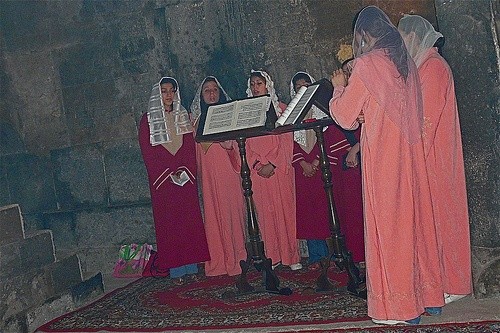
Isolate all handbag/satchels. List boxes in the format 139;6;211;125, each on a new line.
113;244;152;277
142;250;170;277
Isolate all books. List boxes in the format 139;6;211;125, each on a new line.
202;96;271;135
275;84;321;128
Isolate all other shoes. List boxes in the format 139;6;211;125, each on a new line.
172;273;207;285
372;307;441;325
359;261;366;269
308;261;337;269
290;263;302;270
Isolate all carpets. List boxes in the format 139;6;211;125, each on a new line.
266;320;500;333
32;260;433;333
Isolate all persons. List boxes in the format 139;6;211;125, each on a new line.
188;75;250;276
139;77;211;286
244;70;303;271
287;5;474;326
